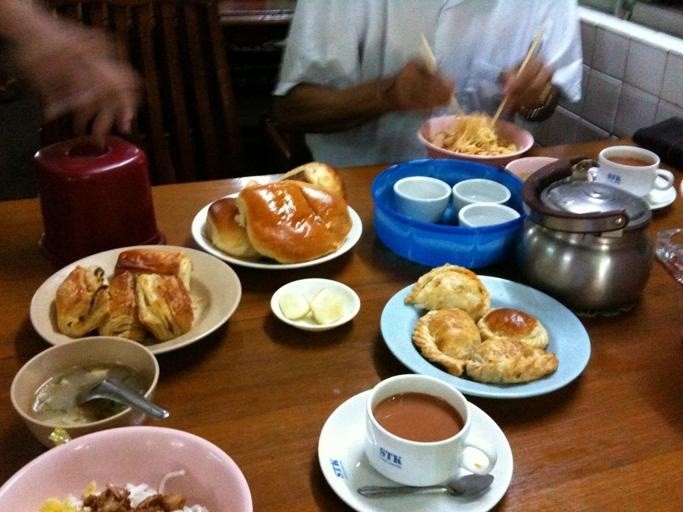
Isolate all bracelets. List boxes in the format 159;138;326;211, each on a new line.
519;86;559;122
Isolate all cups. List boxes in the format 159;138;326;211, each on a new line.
597;144;676;199
364;373;499;489
457;202;520;231
452;177;517;219
391;170;452;224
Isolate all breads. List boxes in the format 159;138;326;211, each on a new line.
57;249;195;345
204;162;353;263
405;263;558;385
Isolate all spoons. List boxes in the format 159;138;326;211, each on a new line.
71;376;170;421
354;474;496;500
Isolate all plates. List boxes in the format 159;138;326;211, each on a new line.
190;191;363;270
269;276;363;333
585;165;678;211
0;425;255;512
379;272;592;401
29;244;242;354
316;388;514;512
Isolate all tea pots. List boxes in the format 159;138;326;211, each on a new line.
514;156;657;321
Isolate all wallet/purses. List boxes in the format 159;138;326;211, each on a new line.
632;116;683;169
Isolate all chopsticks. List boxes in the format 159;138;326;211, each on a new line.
414;35;461;112
488;24;547;131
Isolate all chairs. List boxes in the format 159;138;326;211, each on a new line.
265;41;305;168
45;2;240;183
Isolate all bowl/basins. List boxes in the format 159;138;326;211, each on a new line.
368;158;532;272
7;337;161;449
417;114;537;166
506;157;558;181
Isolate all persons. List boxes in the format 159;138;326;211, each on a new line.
0;0;141;156
270;0;583;169
